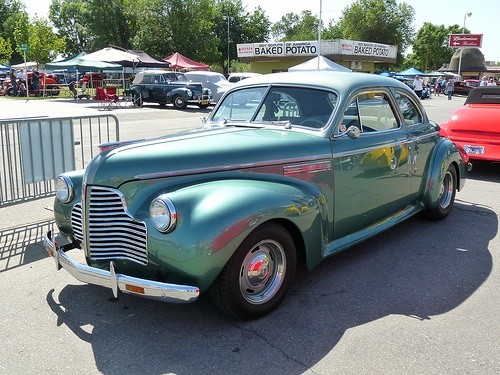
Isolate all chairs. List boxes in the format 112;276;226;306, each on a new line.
278;115;388;134
94;87;122;110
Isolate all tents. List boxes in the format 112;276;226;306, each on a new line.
375;67;461;83
0;45;209;102
287;55;352;73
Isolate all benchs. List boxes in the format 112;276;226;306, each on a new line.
40;88;70;91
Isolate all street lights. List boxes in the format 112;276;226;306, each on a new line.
457;11;473;74
221;15;234;73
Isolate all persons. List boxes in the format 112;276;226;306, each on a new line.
480;76;488;87
425;75;455;101
18;68;26;89
31;67;40;96
10;68;18;96
413;75;424;99
487;79;497;86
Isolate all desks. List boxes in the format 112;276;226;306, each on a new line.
36;84;77;99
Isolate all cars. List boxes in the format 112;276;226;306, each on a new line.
453;77;500;97
208;213;259;257
360;143;410;172
177;71;235;105
42;69;470;321
285;196;328;216
228;73;266;99
439;86;500;165
3;73;61;96
129;70;213;110
